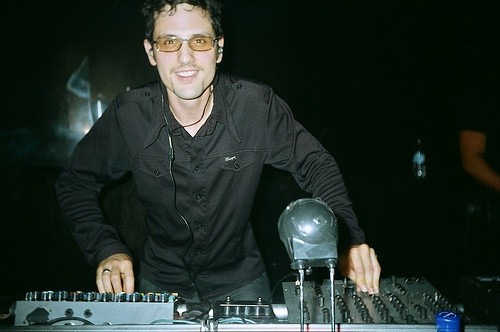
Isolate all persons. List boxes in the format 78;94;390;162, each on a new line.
53;0;381;319
459;71;500;325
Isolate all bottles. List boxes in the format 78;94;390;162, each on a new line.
412;139;426;178
436;312;459;332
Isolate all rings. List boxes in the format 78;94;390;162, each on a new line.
103;269;112;273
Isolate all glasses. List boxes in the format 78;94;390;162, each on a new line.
152;34;220;53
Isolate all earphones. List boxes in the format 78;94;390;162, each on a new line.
149;49;153;56
217;47;223;54
